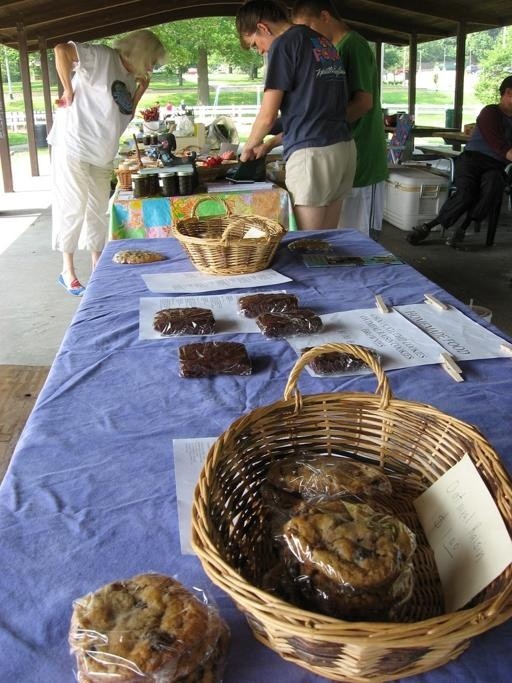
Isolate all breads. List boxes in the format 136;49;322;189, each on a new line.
288;239;329;250
301;345;381;373
262;455;415;621
238;293;298;318
256;308;322;338
154;307;216;335
113;250;166;263
71;574;231;682
178;342;253;377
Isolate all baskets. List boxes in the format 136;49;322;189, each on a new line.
113;158;158;191
171;196;287;276
188;343;511;683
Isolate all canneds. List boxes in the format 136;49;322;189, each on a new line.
143;134;157;145
131;164;193;197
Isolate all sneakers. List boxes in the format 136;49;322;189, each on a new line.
58;272;86;297
404;224;430;245
445;226;465;246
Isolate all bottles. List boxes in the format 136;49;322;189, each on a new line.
143;134;157;145
131;164;194;198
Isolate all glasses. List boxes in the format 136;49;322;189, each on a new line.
250;28;259;51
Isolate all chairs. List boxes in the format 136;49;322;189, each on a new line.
463;122;510;247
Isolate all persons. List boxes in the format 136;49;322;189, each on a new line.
404;76;512;251
291;0;390;239
46;30;166;296
236;0;358;231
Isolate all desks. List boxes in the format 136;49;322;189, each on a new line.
0;177;511;679
384;122;470;151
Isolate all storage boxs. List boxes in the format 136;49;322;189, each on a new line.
383;167;451;232
